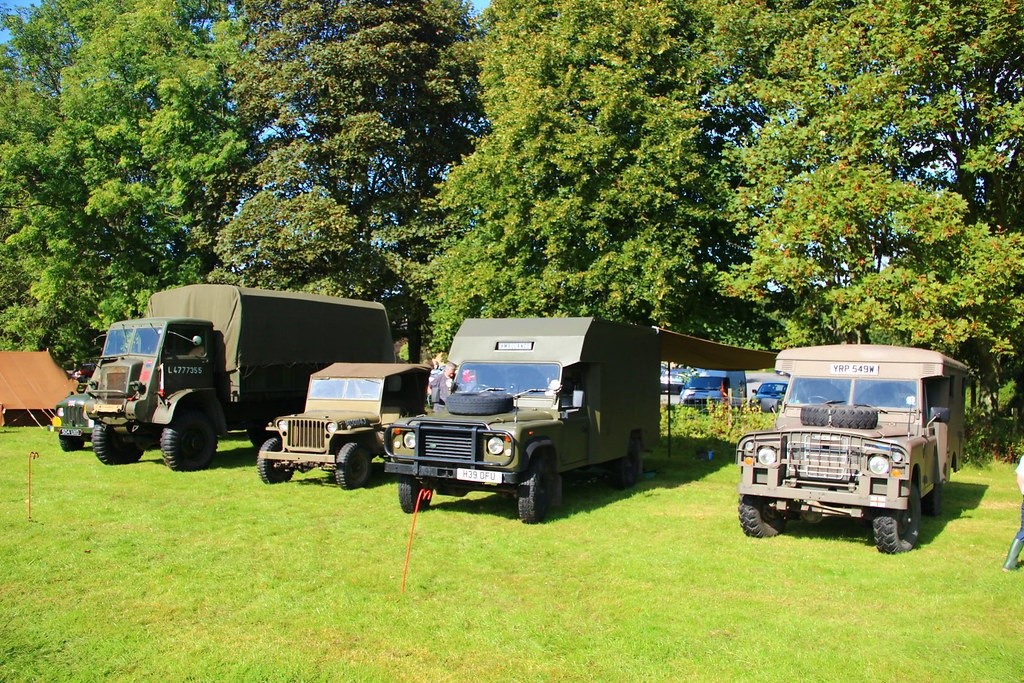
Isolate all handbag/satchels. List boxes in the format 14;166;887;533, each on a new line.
432;381;440;403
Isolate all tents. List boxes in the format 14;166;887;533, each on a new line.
0;348;79;430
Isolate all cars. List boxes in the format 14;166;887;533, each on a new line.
661;363;798;415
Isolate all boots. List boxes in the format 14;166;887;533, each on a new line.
1001;538;1024;573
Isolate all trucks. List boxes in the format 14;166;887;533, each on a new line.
47;282;399;476
51;359;96;451
735;343;972;556
385;314;666;526
258;360;438;491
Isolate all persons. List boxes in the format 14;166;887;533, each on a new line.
427;361;504;411
1001;454;1024;573
